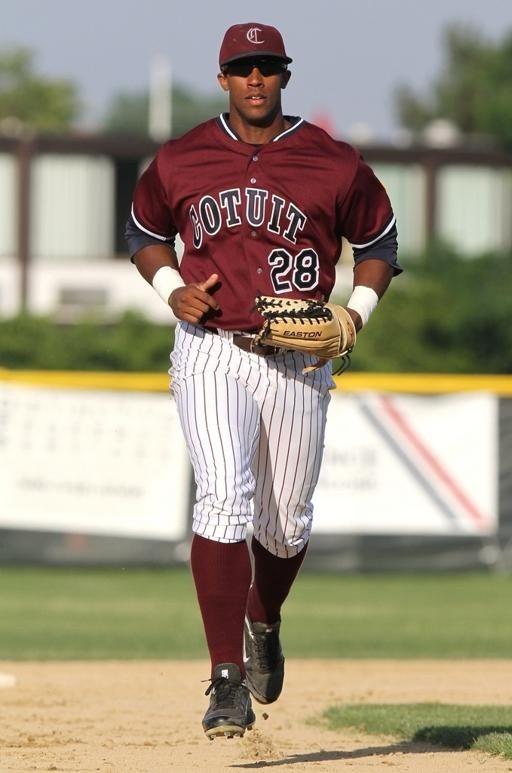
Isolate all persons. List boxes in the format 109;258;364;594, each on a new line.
125;24;404;737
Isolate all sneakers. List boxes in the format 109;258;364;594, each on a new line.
202;663;255;741
244;583;285;704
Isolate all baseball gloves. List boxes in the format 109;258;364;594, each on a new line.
254;295;357;377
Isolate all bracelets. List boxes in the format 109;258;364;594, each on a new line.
348;285;379;327
152;265;186;305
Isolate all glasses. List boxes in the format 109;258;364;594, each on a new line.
223;56;288;77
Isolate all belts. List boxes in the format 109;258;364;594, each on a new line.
199;325;296;357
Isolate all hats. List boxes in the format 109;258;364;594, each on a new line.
219;23;293;67
